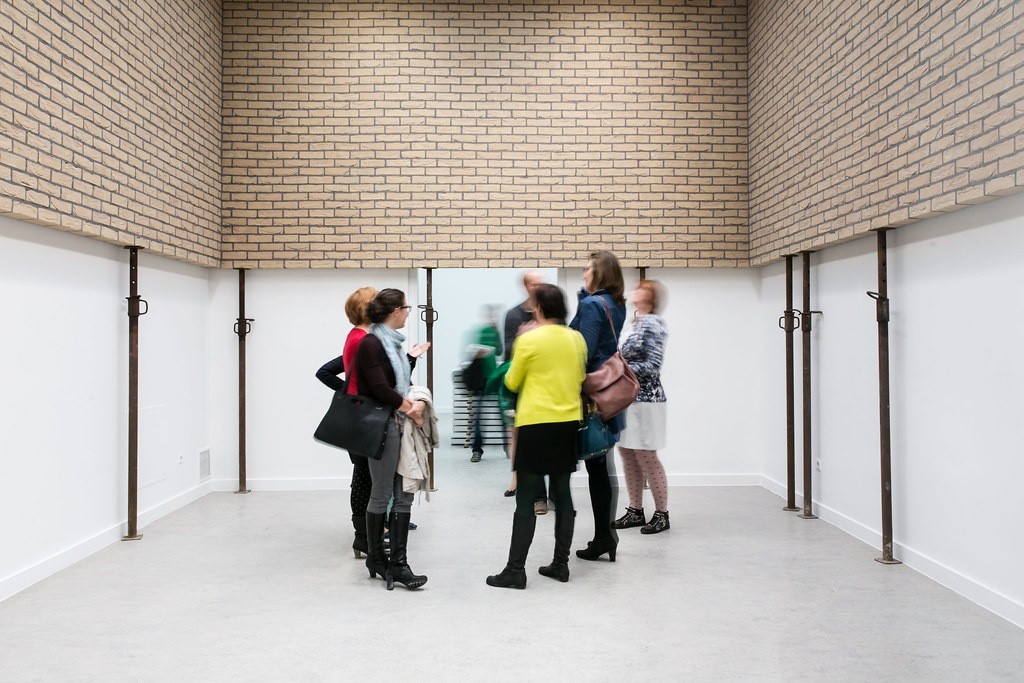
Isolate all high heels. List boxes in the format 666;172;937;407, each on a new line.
575;529;620;563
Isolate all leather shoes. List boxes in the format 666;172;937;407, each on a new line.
505;489;516;496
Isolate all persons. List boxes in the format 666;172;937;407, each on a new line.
486;283;588;588
501;271;555;514
611;279;670;534
356;288;426;590
569;250;617;562
316;287;417;559
469;303;511;463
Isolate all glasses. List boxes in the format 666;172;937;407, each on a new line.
583;266;595;273
389;306;412;314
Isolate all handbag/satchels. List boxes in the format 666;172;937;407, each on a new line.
314;389;394;462
461;357;489;390
583;351;640;422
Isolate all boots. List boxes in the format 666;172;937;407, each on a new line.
386;511;430;590
366;510;390;581
486;513;537;589
353;533;391;559
539;510;576;582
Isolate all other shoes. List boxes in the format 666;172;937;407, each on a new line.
640;510;670;534
534;500;547;514
610;506;646;529
471;452;481;462
